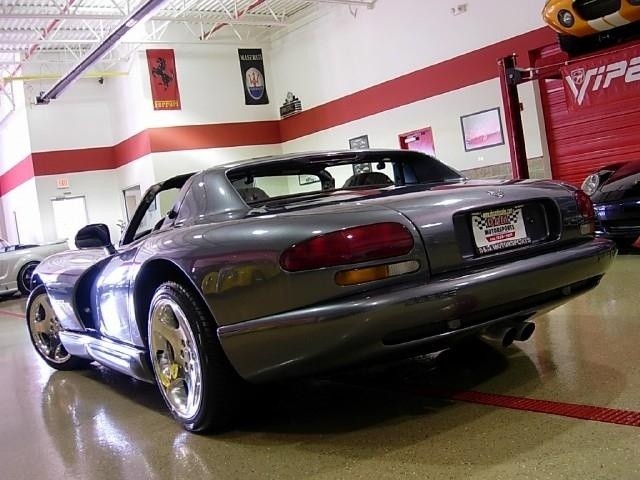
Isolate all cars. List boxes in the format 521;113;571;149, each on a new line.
578;157;638;255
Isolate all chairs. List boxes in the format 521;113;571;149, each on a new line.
235;187;271;206
342;172;396;189
1;245;17;253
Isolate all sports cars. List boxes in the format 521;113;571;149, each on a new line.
0;237;72;300
18;149;606;433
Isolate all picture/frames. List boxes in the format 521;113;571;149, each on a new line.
298;175;320;186
459;106;505;154
348;134;373;176
